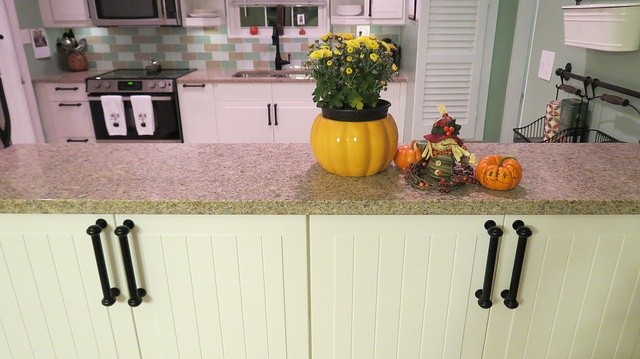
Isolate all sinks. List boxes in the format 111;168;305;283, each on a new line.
233;70;325;80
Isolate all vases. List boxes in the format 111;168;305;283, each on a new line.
312;100;398;176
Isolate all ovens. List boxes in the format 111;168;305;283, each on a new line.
86;93;182;143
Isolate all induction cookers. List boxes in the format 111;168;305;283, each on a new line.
86;68;196;95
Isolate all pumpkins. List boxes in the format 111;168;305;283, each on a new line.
393;143;422;171
475;153;523;191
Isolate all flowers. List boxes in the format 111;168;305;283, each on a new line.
304;32;398;110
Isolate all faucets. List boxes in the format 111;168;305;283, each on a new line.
270;24;291;71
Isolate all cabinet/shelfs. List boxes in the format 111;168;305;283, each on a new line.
180;0;225;27
330;0;405;26
0;214;309;358
39;0;91;28
216;80;321;143
177;83;217;145
309;215;637;358
380;83;406;146
32;82;97;143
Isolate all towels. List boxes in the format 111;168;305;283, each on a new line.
100;95;127;135
130;94;156;137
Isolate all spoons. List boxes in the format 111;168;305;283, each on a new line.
62;36;87;54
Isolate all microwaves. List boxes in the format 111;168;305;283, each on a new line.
88;0;181;27
